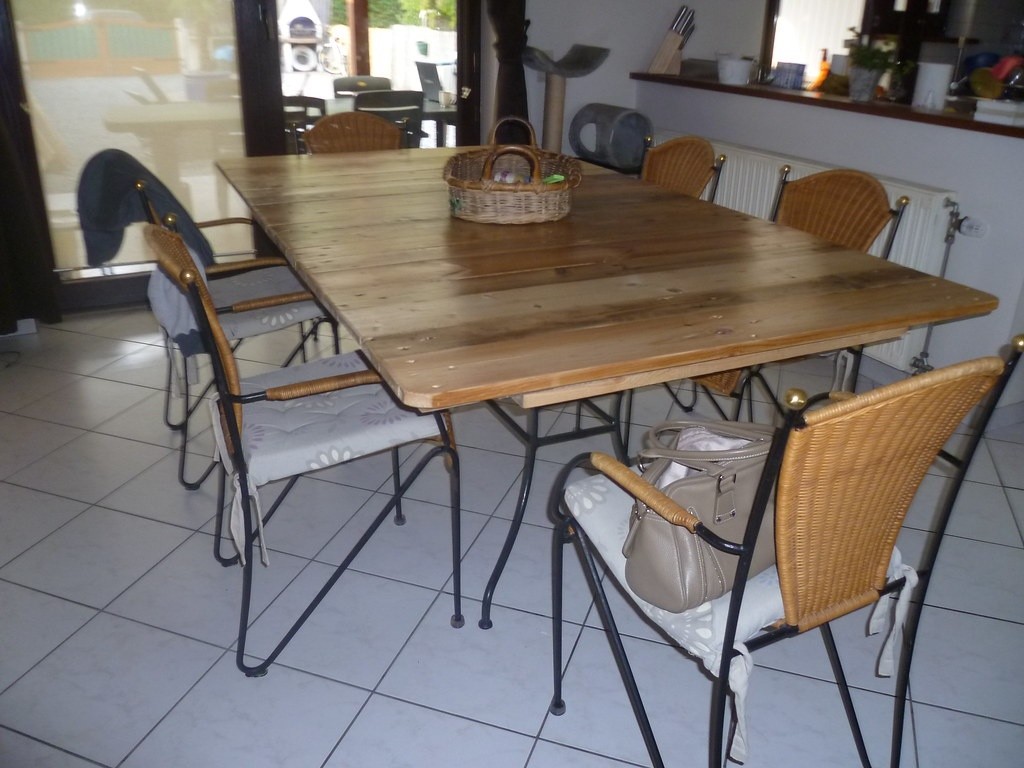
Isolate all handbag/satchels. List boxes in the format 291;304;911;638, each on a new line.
621;420;776;614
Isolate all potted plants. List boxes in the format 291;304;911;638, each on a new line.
849;42;897;103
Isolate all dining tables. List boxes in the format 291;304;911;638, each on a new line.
642;137;910;423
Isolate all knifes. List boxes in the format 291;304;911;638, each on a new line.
669;5;695;51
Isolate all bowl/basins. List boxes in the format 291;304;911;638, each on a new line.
965;51;1024;99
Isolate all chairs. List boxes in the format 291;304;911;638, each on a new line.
75;148;464;679
545;332;1024;768
301;110;403;344
283;76;428;155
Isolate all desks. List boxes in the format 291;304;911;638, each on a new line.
211;144;999;631
284;96;458;148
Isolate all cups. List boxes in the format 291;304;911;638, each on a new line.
438;91;453;108
912;62;954;112
719;57;752;85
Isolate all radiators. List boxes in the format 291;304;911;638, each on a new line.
653;126;987;376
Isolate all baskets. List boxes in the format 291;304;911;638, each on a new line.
444;115;581;225
